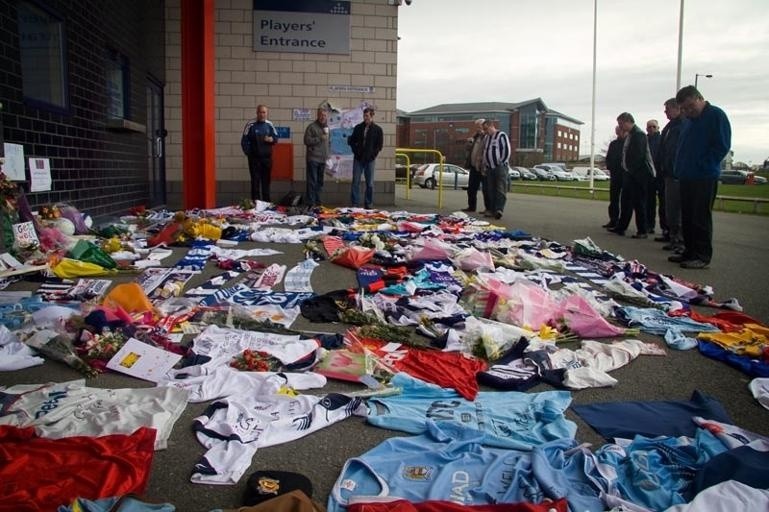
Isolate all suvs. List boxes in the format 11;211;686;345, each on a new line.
533;165;574;181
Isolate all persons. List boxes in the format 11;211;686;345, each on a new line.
241;105;279;202
602;84;731;268
350;108;383;209
460;119;511;219
303;110;330;206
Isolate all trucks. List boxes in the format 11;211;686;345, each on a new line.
573;167;608;181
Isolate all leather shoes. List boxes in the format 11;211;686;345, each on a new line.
654;228;710;269
601;221;654;239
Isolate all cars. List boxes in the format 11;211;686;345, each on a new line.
602;169;611;177
529;168;554;181
409;164;422;179
564;168;590;182
513;167;537;181
694;74;712;90
413;163;470;191
507;166;520;180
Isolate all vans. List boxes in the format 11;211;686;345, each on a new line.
718;170;767;185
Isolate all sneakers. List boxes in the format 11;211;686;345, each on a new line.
462;206;503;219
